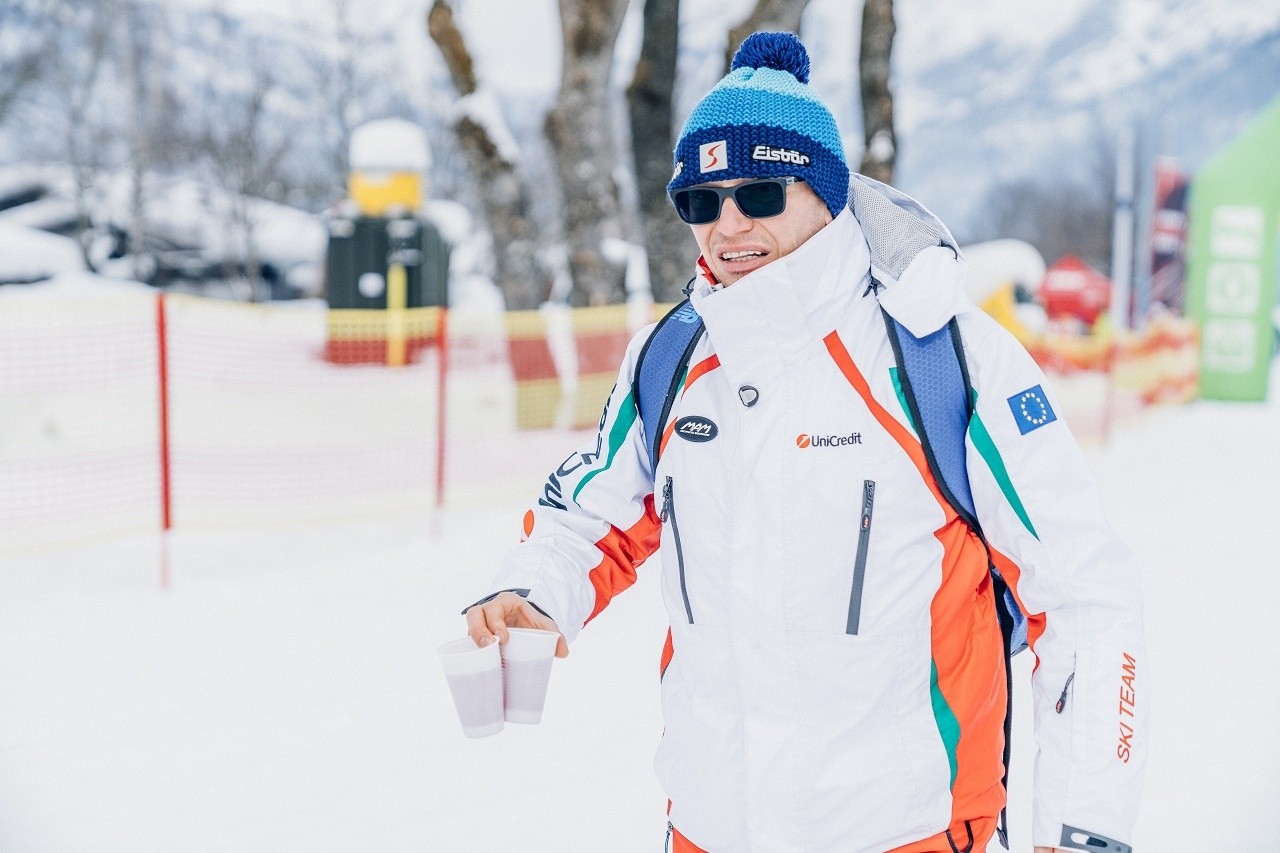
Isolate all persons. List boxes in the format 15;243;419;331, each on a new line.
460;28;1149;852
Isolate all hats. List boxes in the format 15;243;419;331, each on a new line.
666;32;850;219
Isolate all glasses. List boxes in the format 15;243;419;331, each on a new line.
669;173;805;225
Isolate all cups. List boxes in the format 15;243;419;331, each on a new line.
500;625;559;723
436;634;504;737
